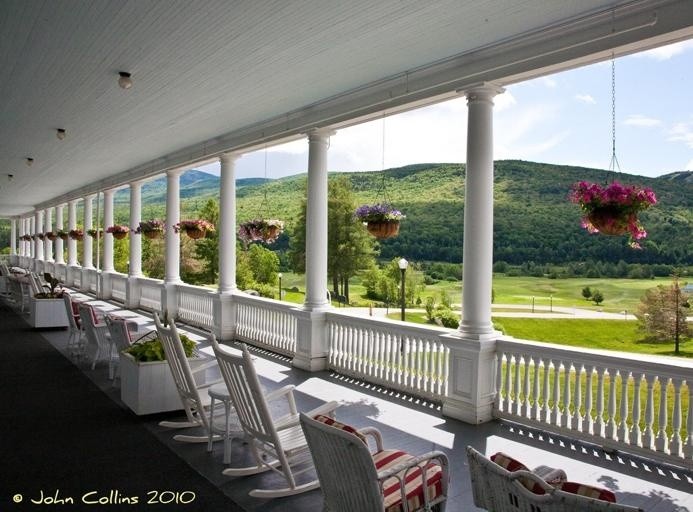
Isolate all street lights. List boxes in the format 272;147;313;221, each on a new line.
279;272;283;300
126;260;130;273
550;294;553;312
532;296;535;313
673;283;681;352
398;258;409;355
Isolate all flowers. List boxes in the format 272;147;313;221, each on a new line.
17;224;129;240
568;175;658;250
238;219;284;245
132;216;165;237
356;203;405;222
173;218;215;232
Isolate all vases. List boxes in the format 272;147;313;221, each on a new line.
143;230;158;238
186;230;205;239
586;216;626;236
367;221;399;238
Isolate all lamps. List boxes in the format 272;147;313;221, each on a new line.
56;128;66;138
25;157;33;167
117;70;131;89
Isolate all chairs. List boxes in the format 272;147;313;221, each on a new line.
0;259;637;512
